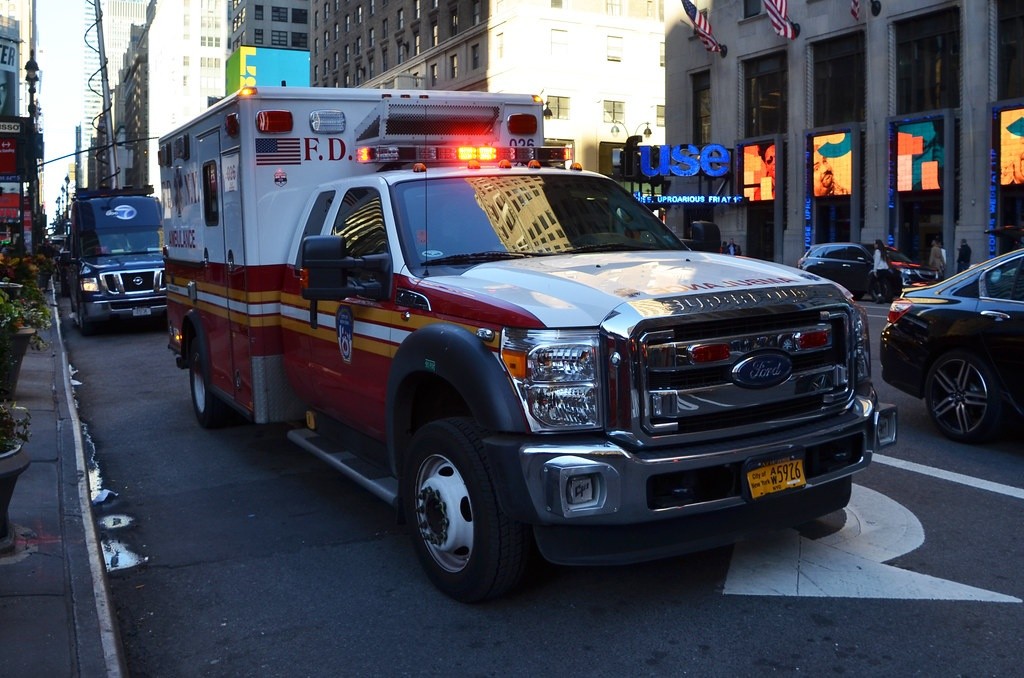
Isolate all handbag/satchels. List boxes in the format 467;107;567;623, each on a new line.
876;287;890;304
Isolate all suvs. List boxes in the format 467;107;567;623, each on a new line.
796;242;944;305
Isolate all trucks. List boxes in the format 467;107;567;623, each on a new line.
70;187;167;336
157;84;900;607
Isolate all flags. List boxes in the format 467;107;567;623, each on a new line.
681;0;725;56
851;0;862;20
765;0;797;41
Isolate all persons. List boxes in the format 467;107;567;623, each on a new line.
957;239;971;274
754;143;777;200
936;240;946;263
929;239;945;273
720;238;742;256
814;145;850;196
873;238;890;304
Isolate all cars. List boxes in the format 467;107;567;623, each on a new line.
880;248;1024;447
